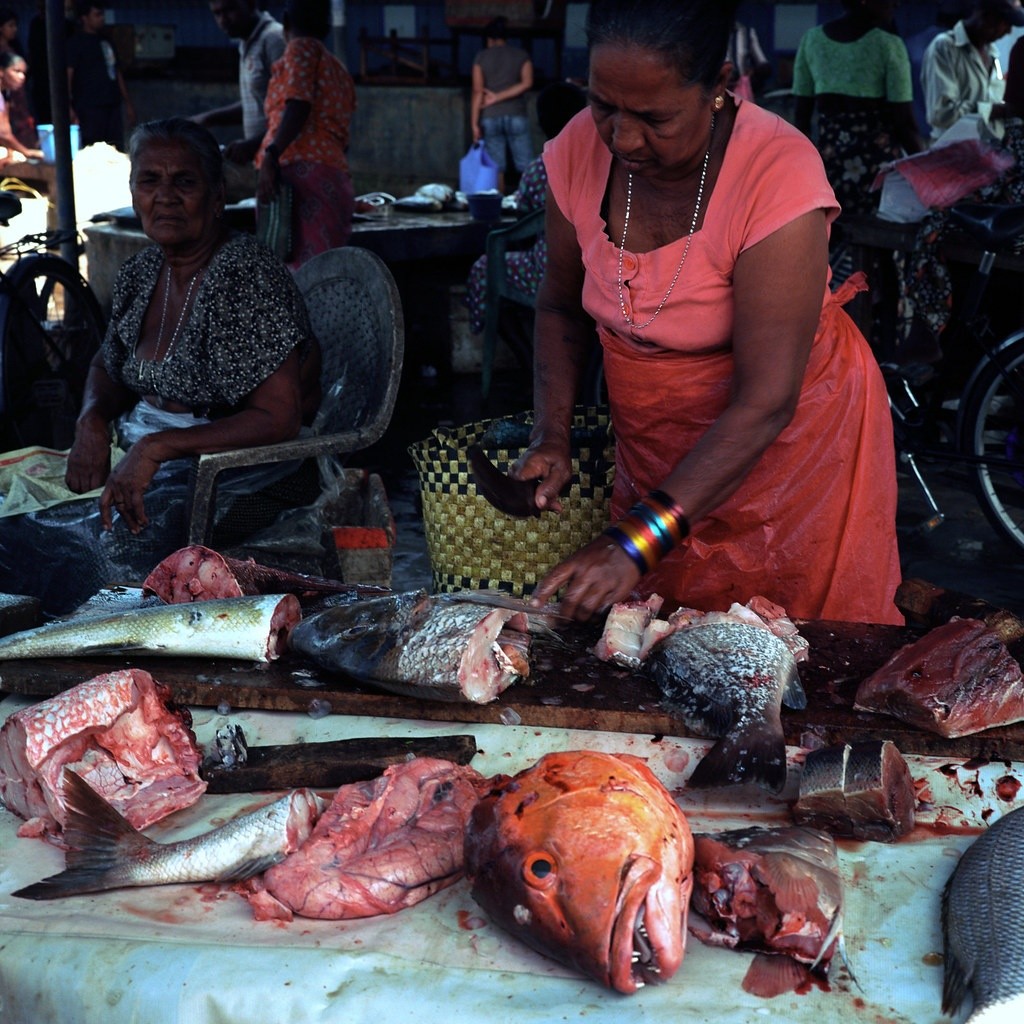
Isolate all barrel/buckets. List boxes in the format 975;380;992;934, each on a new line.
36;123;80;164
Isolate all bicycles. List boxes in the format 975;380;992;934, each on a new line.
937;193;1024;562
0;191;108;453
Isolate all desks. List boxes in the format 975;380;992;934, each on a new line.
840;222;1024;334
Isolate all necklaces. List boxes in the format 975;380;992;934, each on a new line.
618;113;715;329
151;267;202;409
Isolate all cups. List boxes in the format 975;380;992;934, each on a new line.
466;193;503;221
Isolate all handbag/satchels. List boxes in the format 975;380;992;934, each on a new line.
255;180;297;258
459;139;501;195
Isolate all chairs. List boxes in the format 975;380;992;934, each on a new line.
480;205;547;400
187;244;406;550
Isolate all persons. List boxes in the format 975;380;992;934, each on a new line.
466;82;587;336
471;16;534;196
66;0;136;152
184;0;288;163
0;52;45;170
0;117;328;620
790;0;953;365
919;0;1024;145
0;10;34;134
254;0;358;275
506;0;906;630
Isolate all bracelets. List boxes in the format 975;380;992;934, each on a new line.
601;489;690;576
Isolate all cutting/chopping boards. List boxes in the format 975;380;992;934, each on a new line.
0;586;1023;760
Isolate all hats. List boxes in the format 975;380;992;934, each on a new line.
975;0;1024;27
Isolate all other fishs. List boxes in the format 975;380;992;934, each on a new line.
0;542;1024;1024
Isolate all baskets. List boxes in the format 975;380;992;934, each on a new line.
410;405;617;605
